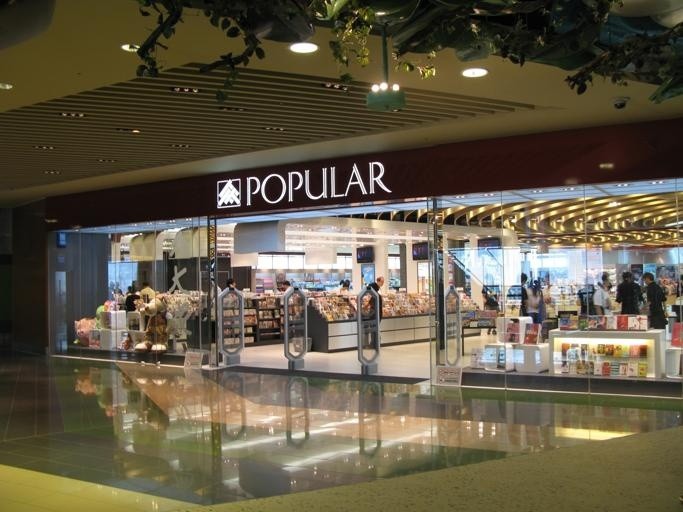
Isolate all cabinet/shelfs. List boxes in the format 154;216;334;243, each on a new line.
309;295;481;349
547;327;668;381
214;298;304;349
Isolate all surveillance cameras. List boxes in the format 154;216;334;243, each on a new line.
611;96;631;109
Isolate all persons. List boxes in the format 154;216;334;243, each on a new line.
107;279;136;302
365;281;382;350
515;271;682;337
124;287;138;311
335;279;352;294
139;280;155;328
225;278;236;287
372;275;385;292
282;281;294;296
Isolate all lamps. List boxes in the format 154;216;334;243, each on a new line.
363;25;405;114
306;246;337;265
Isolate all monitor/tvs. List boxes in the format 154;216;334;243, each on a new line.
477;238;500;257
412;243;428;260
356;247;372;263
